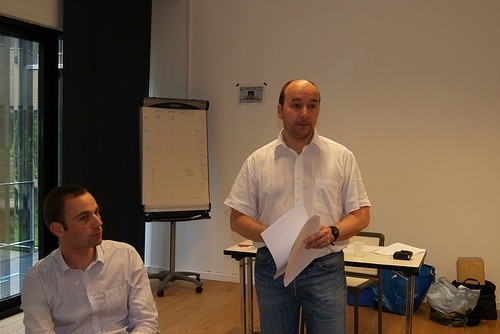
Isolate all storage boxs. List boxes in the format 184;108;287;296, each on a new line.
456;256;486;284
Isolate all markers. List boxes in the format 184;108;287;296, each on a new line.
191;215;201;219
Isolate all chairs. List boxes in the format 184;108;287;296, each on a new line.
301;231;385;334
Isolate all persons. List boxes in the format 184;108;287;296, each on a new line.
20;185;160;334
224;79;370;334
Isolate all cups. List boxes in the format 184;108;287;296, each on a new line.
353;242;364;257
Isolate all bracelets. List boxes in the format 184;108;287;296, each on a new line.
329;225;340;245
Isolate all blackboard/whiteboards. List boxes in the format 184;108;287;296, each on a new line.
136;97;212;217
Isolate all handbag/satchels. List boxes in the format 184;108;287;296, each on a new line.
451;278;498;320
427;275;481;327
371;262;436;316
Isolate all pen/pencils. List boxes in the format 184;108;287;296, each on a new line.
237;244;253;247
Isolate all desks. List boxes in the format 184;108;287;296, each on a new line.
224;239;427;334
